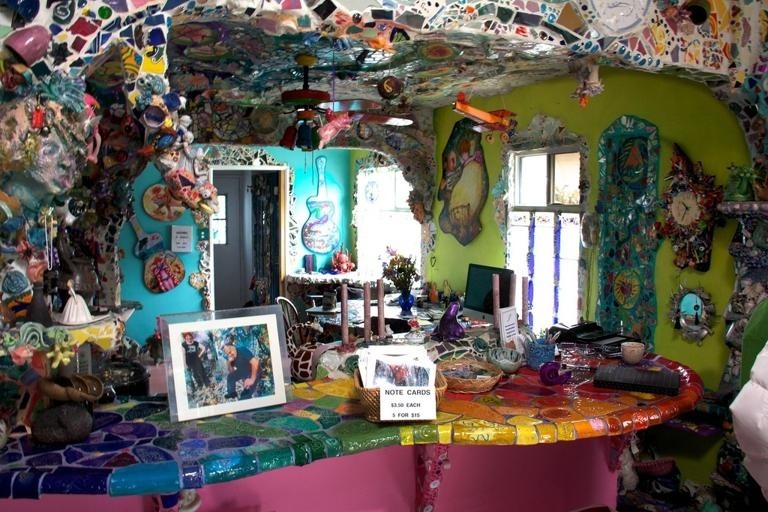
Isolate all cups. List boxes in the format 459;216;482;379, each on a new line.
527;340;555;371
305;254;313;273
620;341;650;365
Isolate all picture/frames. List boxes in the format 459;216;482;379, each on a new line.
157;305;293;423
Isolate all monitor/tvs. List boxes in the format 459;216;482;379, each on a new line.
462;263;514;337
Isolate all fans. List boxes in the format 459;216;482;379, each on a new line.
227;57;413;127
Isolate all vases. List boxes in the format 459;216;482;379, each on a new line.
399;290;414;315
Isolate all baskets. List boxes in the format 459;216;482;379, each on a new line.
353;367;447;424
439;359;503;394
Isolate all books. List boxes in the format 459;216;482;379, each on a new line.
589;363;682;398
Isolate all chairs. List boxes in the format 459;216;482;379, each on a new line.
305;286;365;306
275;296;303;346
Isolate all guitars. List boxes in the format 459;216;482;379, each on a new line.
301;156;341;254
127;203;185;294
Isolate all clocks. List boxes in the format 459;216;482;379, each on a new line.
659;144;721;271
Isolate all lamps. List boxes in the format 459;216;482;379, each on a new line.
281;107;325;151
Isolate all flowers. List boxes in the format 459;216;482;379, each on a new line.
382;244;420;304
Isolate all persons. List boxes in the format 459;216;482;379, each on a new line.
181;332;210;389
223;342;262;399
